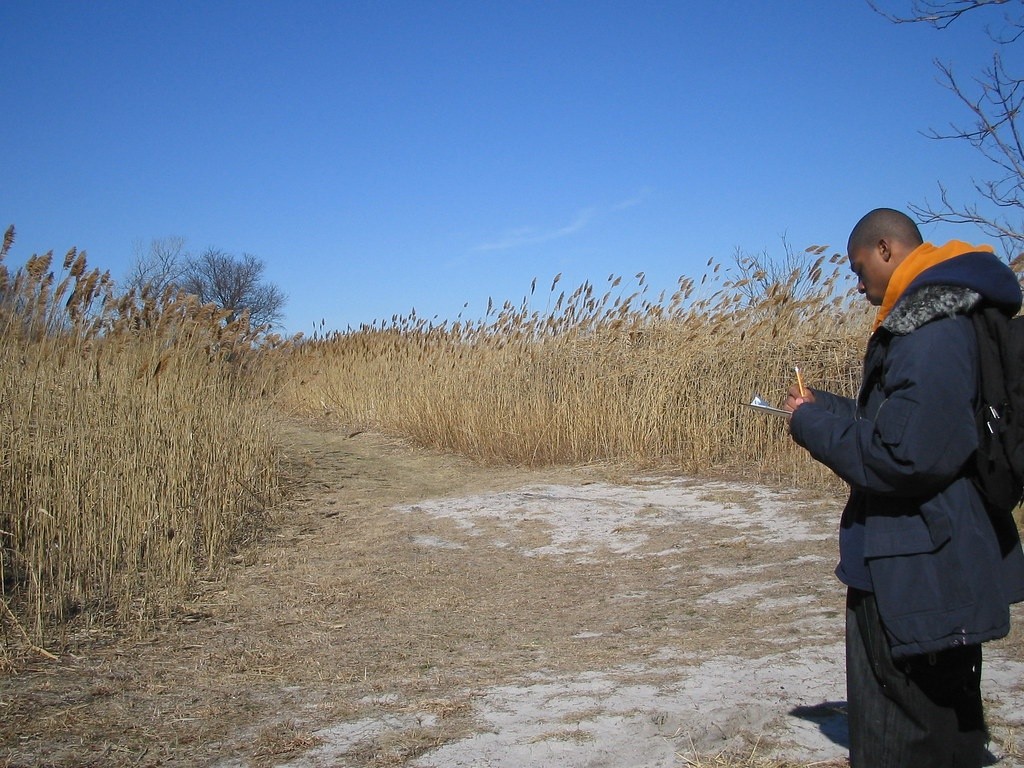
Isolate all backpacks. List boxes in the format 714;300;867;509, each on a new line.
962;299;1024;519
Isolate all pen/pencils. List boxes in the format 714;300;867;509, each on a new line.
794;366;804;397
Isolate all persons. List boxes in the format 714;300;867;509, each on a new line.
781;208;1024;768
